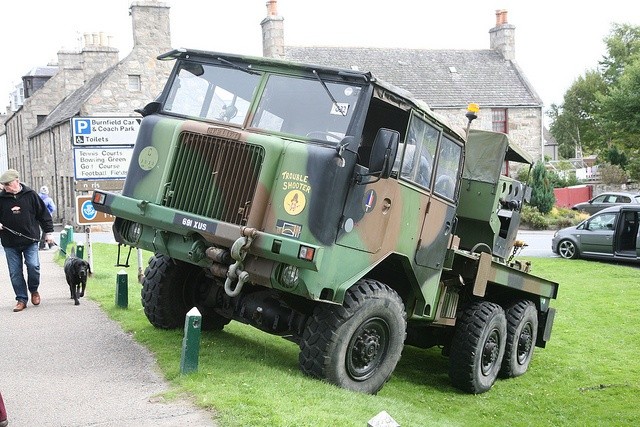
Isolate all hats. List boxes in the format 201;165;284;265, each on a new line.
39;185;50;195
1;169;19;182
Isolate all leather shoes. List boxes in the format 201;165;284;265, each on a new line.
31;290;40;305
12;300;27;312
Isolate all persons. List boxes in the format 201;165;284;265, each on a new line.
34;185;57;251
0;168;57;313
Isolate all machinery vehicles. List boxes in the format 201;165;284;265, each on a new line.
91;48;559;395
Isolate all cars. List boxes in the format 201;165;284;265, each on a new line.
552;205;640;266
571;192;640;215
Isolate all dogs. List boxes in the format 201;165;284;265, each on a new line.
64;254;92;304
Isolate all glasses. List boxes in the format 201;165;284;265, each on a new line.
1;182;11;187
